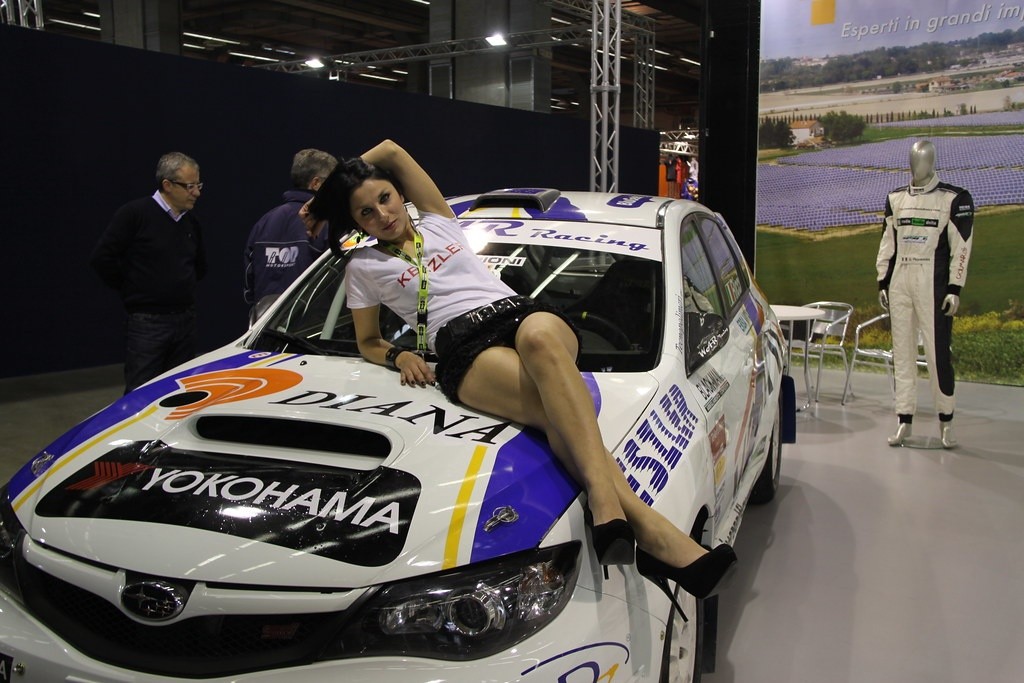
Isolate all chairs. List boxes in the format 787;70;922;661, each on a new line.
841;311;927;405
778;301;857;402
591;258;663;357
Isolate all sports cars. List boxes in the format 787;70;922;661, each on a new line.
0;184;798;683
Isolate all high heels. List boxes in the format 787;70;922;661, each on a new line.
582;499;635;581
634;543;739;622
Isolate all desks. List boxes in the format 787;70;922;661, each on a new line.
769;304;826;411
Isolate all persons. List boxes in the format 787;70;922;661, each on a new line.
875;141;974;447
85;153;207;397
244;150;338;321
299;140;737;599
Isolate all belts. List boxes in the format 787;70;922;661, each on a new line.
446;293;534;342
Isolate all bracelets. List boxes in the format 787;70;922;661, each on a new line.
386;347;412;370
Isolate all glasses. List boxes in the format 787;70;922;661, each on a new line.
160;178;204;191
